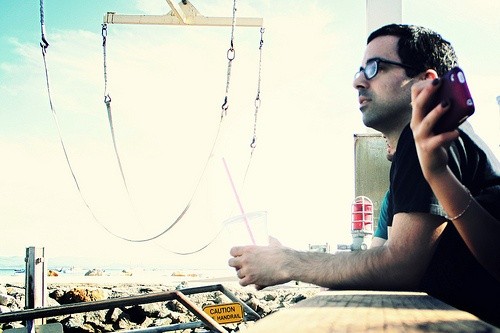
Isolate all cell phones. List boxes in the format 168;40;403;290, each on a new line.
422;66;475;136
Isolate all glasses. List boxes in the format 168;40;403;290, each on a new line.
354;58;406;80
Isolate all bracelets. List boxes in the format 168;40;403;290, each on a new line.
445;192;472;220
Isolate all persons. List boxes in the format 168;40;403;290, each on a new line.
409;78;500;279
228;22;500;328
